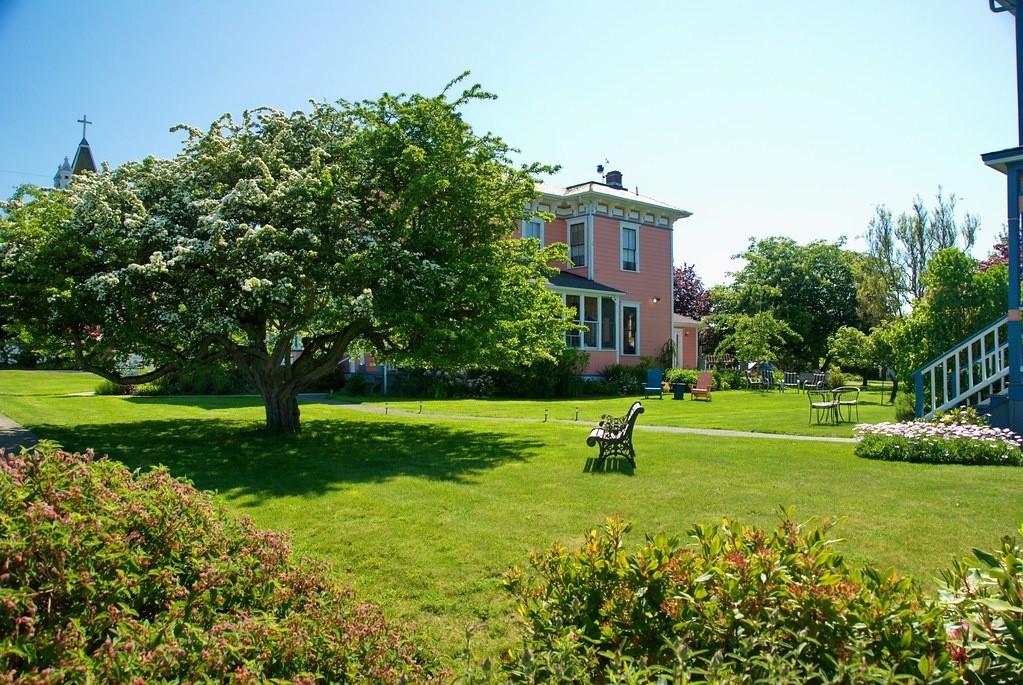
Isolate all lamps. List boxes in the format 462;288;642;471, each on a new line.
653;298;660;303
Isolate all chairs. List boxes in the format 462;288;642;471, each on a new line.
803;373;826;394
689;372;713;401
807;386;860;424
641;368;665;399
761;370;781;393
781;372;800;394
746;370;762;390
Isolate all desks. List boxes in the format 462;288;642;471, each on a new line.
672;383;686;399
811;390;851;426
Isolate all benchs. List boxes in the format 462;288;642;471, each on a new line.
586;402;645;470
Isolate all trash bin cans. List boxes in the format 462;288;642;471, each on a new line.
671;383;686;399
